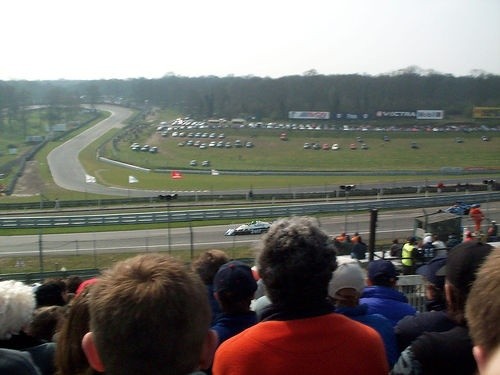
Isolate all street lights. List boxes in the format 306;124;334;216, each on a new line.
483;179;496;234
156;193;178;255
339;184;357;234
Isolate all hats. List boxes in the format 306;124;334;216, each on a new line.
327;261;364;300
464;231;471;235
366;260;400;284
435;240;496;288
406;236;417;241
415;255;450;284
423;236;432;243
214;261;258;304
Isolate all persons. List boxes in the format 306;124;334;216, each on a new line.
326;256;417;369
192;249;227;283
488;221;497;242
391;231;473;292
210;261;258;342
466;246;500;375
394;252;450;352
212;218;390;375
333;232;368;260
0;277;101;375
82;253;218;375
470;204;484;231
391;241;496;375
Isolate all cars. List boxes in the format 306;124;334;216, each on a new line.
224;220;272;236
128;114;500;167
444;201;481;216
